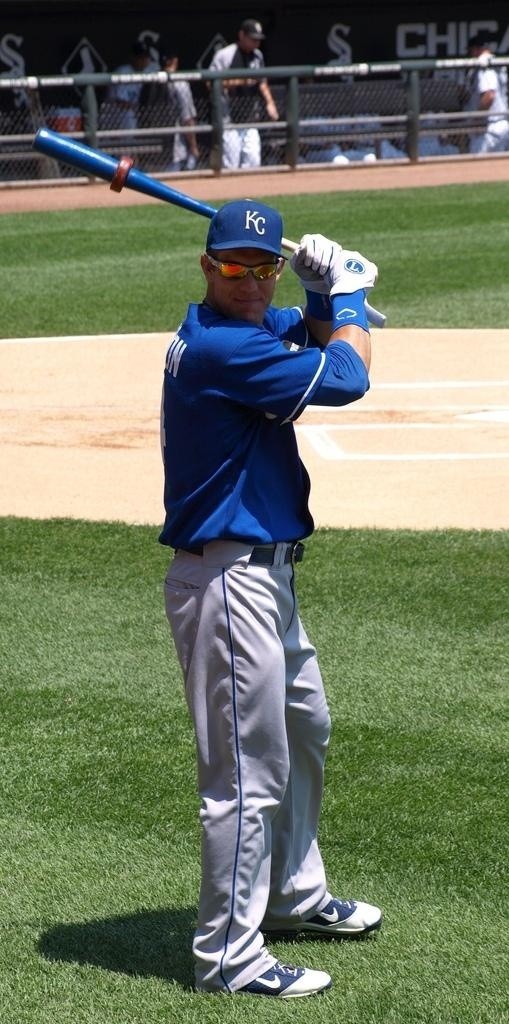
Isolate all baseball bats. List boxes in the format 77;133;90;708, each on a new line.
33;125;298;254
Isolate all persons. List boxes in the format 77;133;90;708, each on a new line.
458;33;508;153
105;42;200;173
206;20;279;169
158;198;384;998
260;107;459;166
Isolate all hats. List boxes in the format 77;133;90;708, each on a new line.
206;200;288;259
242;20;264;39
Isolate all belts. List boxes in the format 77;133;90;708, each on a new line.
182;540;304;564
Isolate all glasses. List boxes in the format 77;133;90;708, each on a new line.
204;253;281;280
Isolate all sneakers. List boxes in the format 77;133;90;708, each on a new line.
236;962;330;998
260;898;381;936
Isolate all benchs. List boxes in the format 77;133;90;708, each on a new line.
100;80;486;165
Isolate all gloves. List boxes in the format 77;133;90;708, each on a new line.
290;234;377;300
